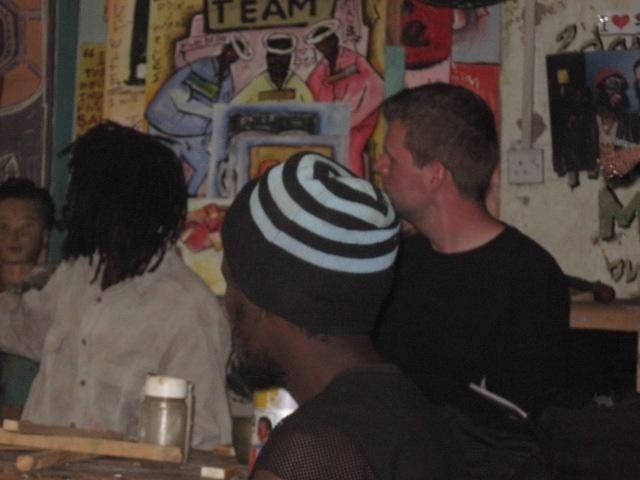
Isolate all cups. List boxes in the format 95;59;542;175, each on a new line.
136;374;188;469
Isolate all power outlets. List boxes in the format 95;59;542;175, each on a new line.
505;148;545;184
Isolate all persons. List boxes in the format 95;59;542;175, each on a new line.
140;32;253;201
303;20;389;183
226;29;318;105
374;78;574;438
1;120;237;457
564;70;638;174
220;148;476;480
1;174;56;414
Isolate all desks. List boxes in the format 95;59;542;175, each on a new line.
566;298;639;393
0;418;251;480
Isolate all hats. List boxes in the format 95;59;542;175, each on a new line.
594;67;628;92
220;151;401;326
